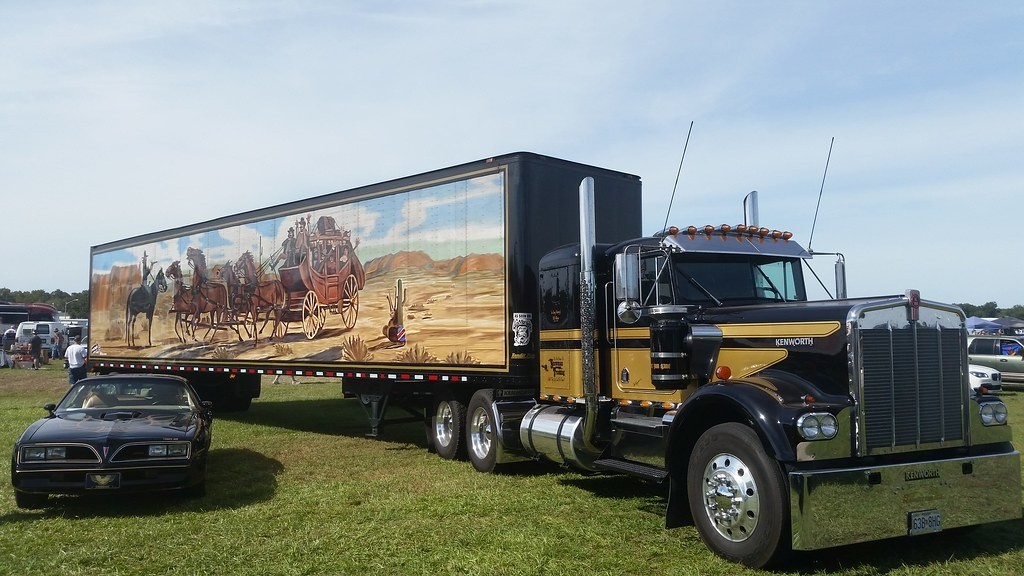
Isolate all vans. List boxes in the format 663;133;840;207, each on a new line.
967;335;1024;384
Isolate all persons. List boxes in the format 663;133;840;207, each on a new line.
28;330;42;370
967;328;1024;337
272;374;301;385
51;328;63;361
63;334;88;385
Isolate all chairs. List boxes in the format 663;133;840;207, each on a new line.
81;393;122;409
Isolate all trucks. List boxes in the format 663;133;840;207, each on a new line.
0;302;59;324
14;319;88;358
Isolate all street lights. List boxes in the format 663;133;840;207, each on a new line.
65;299;78;319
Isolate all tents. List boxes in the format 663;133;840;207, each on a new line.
991;316;1023;330
966;316;1002;337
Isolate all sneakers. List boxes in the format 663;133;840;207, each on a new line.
273;381;281;386
292;381;301;385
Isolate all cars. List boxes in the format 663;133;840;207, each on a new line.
968;364;1002;394
11;373;214;509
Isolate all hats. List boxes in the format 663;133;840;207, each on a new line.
32;330;39;334
53;328;59;331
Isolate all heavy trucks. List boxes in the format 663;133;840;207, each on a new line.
87;120;1024;570
